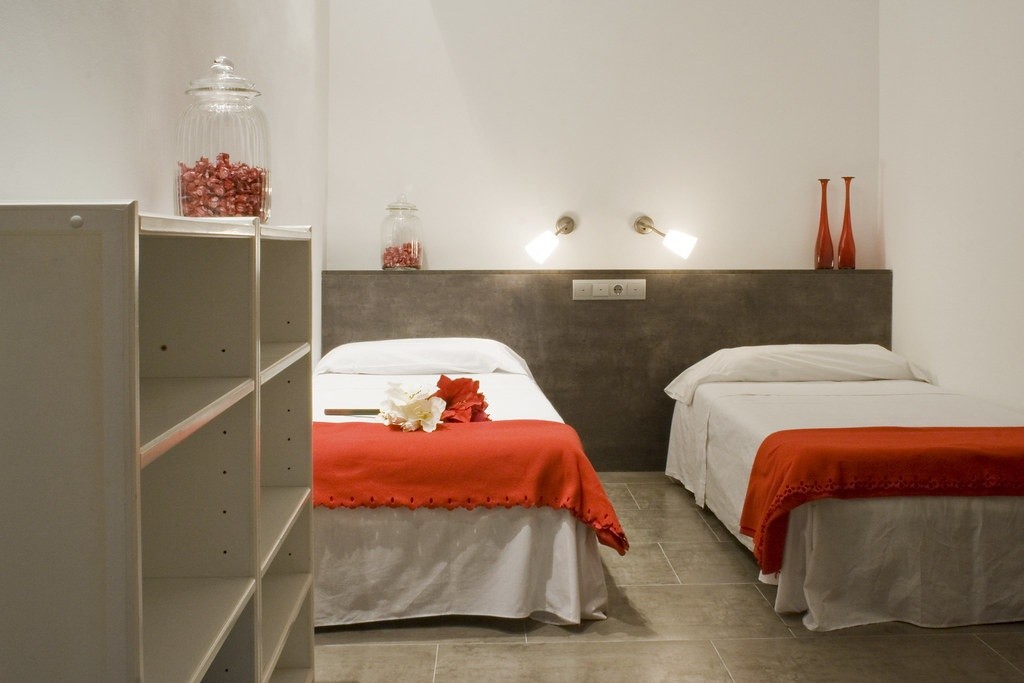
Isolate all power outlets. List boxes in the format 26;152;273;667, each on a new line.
610;279;627;300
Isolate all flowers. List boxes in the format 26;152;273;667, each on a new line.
325;375;492;432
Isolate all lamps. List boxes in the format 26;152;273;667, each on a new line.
634;216;698;260
525;216;574;264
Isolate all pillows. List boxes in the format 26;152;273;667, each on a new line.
313;338;525;377
663;344;932;407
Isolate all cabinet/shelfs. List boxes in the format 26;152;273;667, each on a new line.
0;201;315;683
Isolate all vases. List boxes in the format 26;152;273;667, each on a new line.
814;177;856;269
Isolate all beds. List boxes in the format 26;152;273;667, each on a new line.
313;370;631;626
665;380;1024;633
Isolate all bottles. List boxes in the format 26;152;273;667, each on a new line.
174;56;272;219
378;194;422;270
